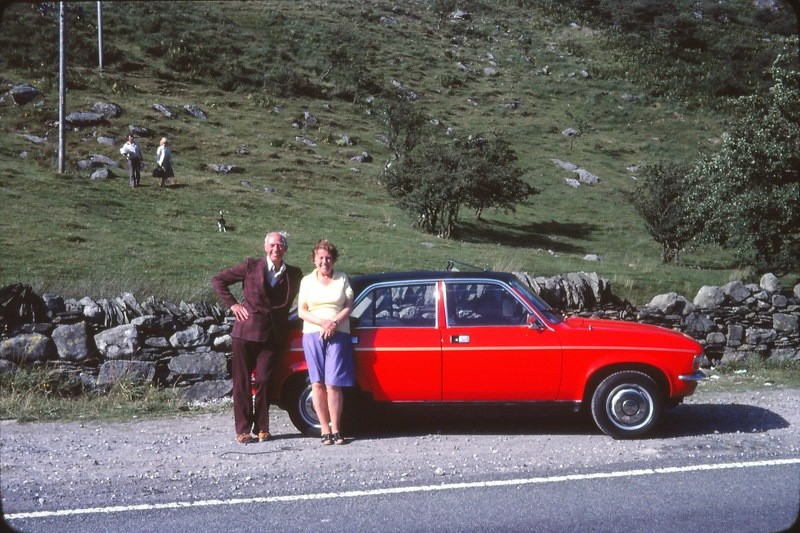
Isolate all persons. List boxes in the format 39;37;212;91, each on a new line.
211;232;304;442
298;239;354;445
157;137;174;188
123;134;144;189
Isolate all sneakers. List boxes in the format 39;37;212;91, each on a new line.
236;432;252;443
252;428;271;441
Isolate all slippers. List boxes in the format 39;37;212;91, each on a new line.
321;434;332;445
332;433;343;445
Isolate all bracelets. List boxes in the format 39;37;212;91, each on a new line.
334;319;340;325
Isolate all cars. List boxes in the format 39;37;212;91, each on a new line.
250;269;707;441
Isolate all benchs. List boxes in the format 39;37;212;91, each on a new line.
360;316;404;326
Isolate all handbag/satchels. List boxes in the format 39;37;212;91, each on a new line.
153;166;164;178
